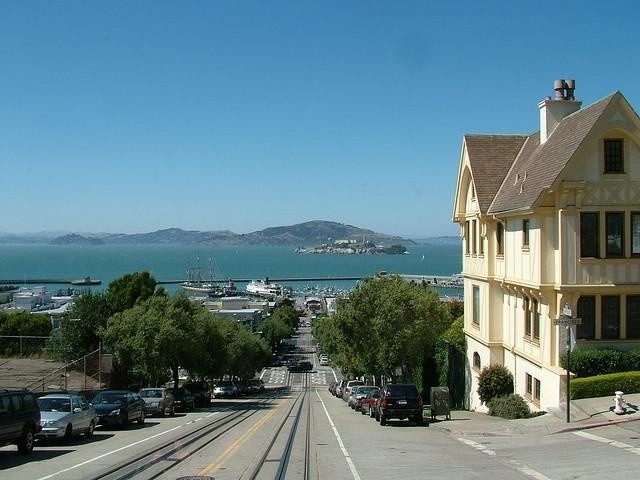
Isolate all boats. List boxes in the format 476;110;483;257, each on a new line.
72;277;101;285
246;277;283;296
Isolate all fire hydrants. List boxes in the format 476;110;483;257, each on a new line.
614;391;624;414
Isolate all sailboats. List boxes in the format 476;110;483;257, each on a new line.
181;246;237;298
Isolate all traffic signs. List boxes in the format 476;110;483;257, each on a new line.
552;303;582;326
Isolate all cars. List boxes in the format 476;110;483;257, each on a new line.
287;361;313;373
0;387;42;455
329;378;422;427
247;379;264;393
36;381;242;444
315;343;332;367
